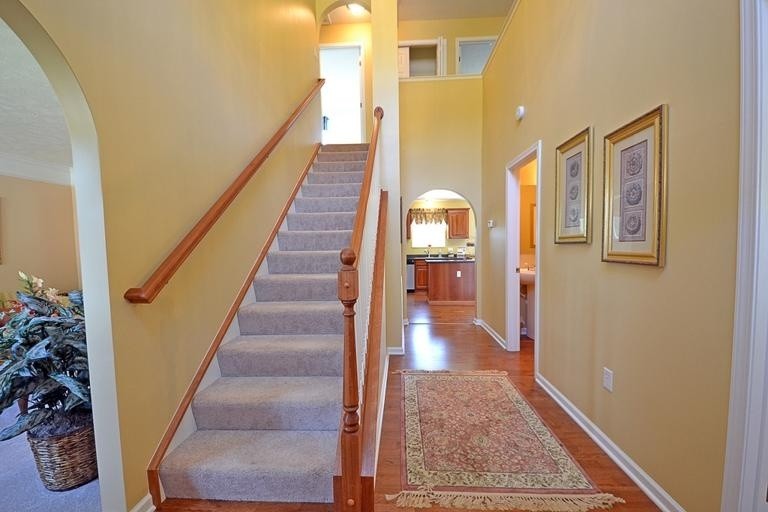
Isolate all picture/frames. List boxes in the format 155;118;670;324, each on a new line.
552;101;673;269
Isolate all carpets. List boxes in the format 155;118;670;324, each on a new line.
159;142;368;505
384;367;627;512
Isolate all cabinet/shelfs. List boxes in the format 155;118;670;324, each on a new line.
415;261;429;288
448;210;469;239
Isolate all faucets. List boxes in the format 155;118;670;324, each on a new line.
424;247;431;257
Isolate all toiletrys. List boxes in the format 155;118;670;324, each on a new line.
437;248;442;257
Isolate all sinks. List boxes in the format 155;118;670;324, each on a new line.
520;269;535;285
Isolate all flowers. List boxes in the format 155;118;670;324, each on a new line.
1;269;95;445
